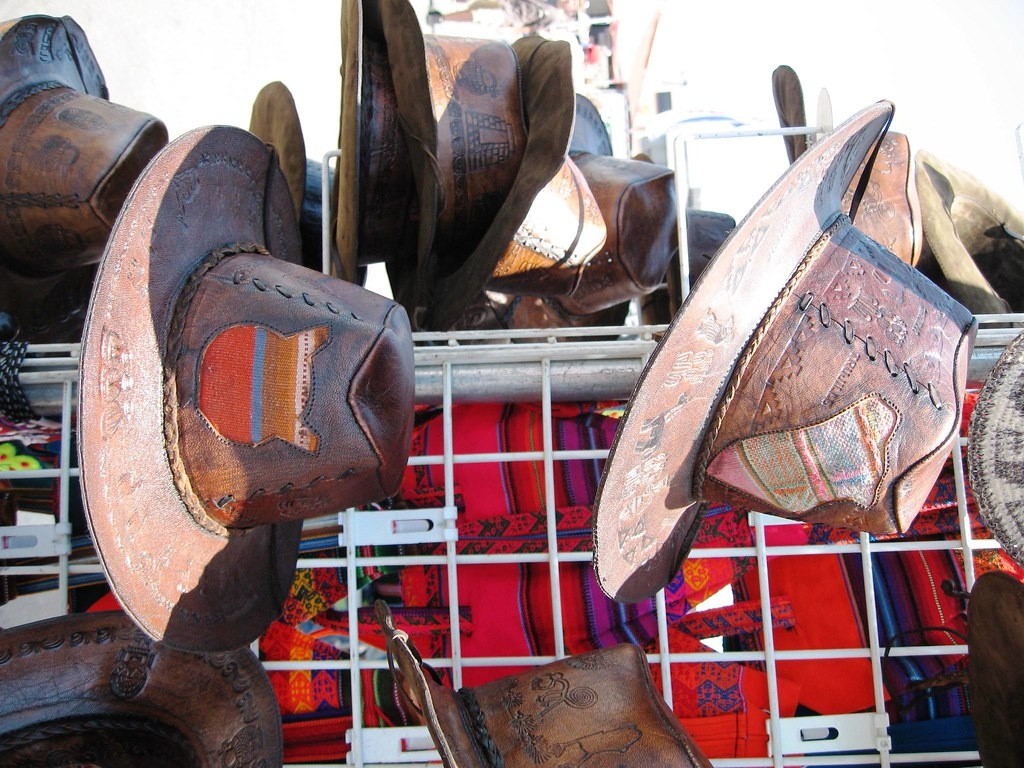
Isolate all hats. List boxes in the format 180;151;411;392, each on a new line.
0;14;172;342
375;599;712;768
591;101;979;605
0;611;283;768
969;332;1024;570
338;1;737;344
78;125;417;654
966;572;1024;768
249;81;367;288
